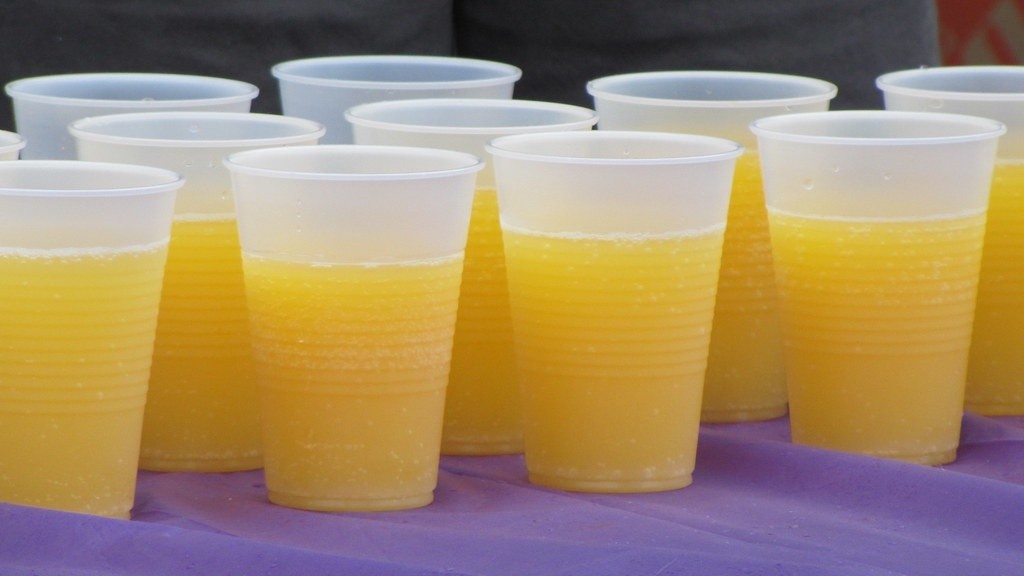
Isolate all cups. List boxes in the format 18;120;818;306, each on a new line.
0;51;1024;527
